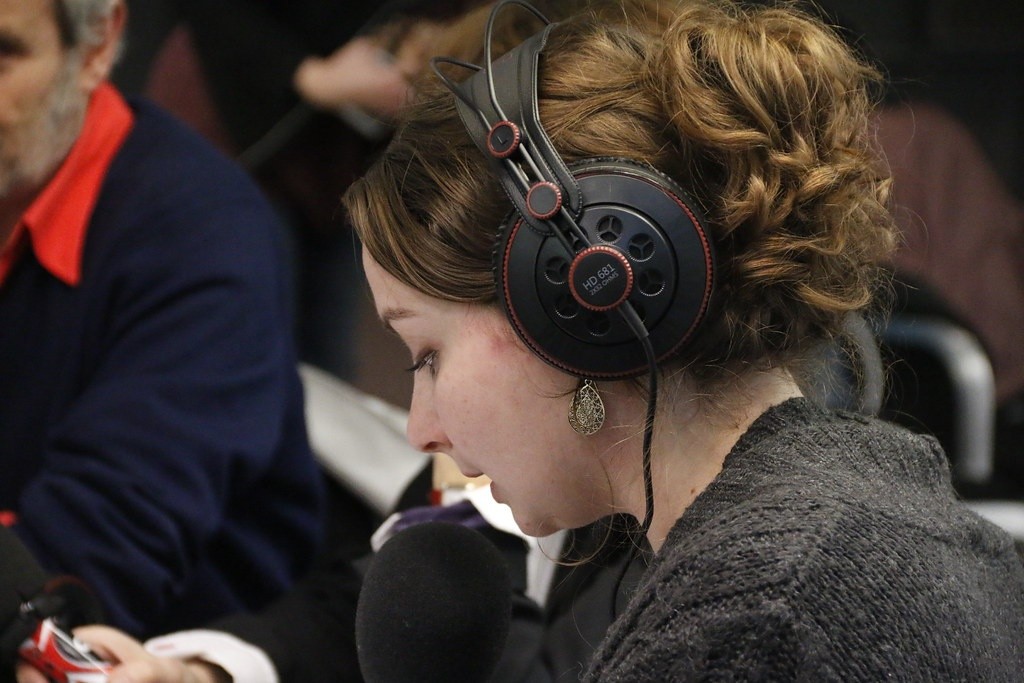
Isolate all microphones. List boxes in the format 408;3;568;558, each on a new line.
357;523;511;683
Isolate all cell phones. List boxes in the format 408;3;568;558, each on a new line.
20;616;114;683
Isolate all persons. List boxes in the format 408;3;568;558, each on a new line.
0;3;1018;683
342;1;1017;682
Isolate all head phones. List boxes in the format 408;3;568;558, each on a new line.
426;0;716;380
